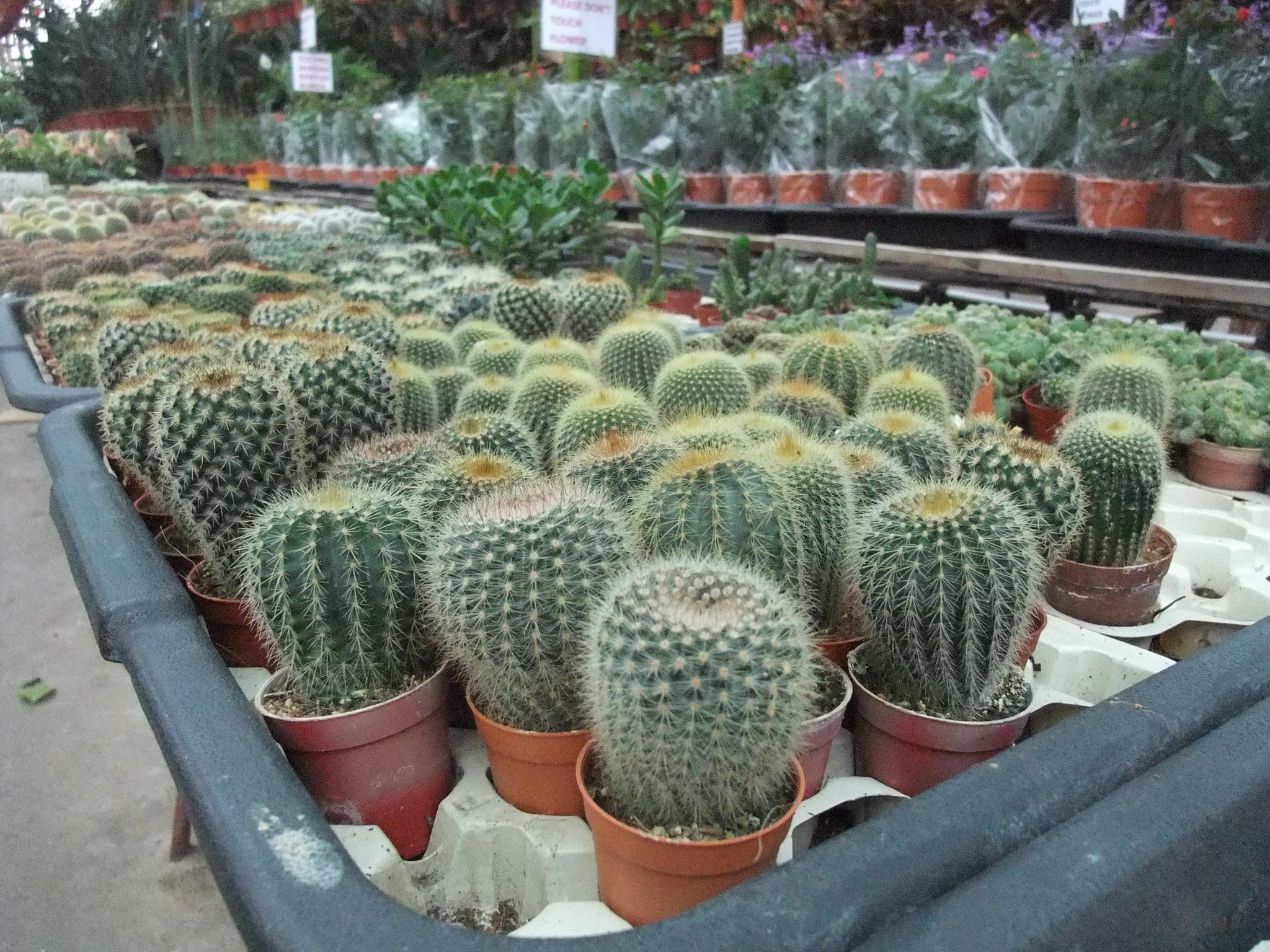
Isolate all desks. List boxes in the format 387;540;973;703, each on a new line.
33;391;1270;952
768;233;1270;346
603;220;767;268
0;293;101;411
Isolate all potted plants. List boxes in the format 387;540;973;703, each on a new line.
0;0;1270;925
